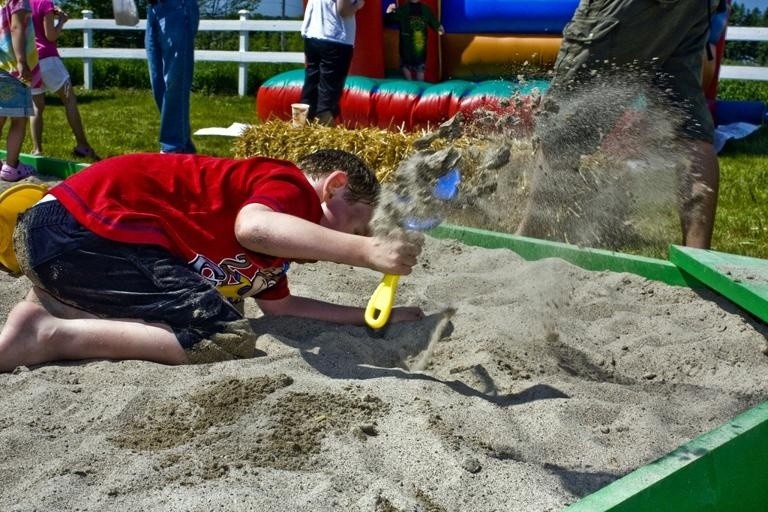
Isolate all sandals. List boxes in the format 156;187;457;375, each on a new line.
73;145;101;160
0;160;34;181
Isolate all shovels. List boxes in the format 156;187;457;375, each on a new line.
364;168;460;329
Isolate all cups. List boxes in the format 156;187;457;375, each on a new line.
289;101;310;127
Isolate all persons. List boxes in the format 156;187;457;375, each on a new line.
144;0;199;153
0;0;102;181
514;0;720;249
0;149;424;373
384;0;445;81
298;0;365;120
